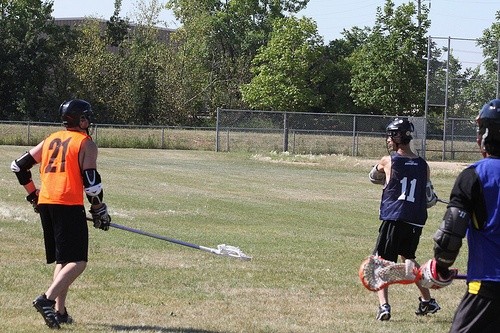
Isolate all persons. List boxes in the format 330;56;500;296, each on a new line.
368;118;440;320
12;100;111;329
417;98;499;333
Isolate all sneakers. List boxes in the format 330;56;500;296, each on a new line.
54;307;73;325
32;292;60;330
415;295;440;316
376;302;391;321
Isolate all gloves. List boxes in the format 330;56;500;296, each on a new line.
26;189;40;213
419;259;458;289
89;204;111;231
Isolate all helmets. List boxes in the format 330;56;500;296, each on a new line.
474;99;500;156
386;117;414;143
58;99;92;128
